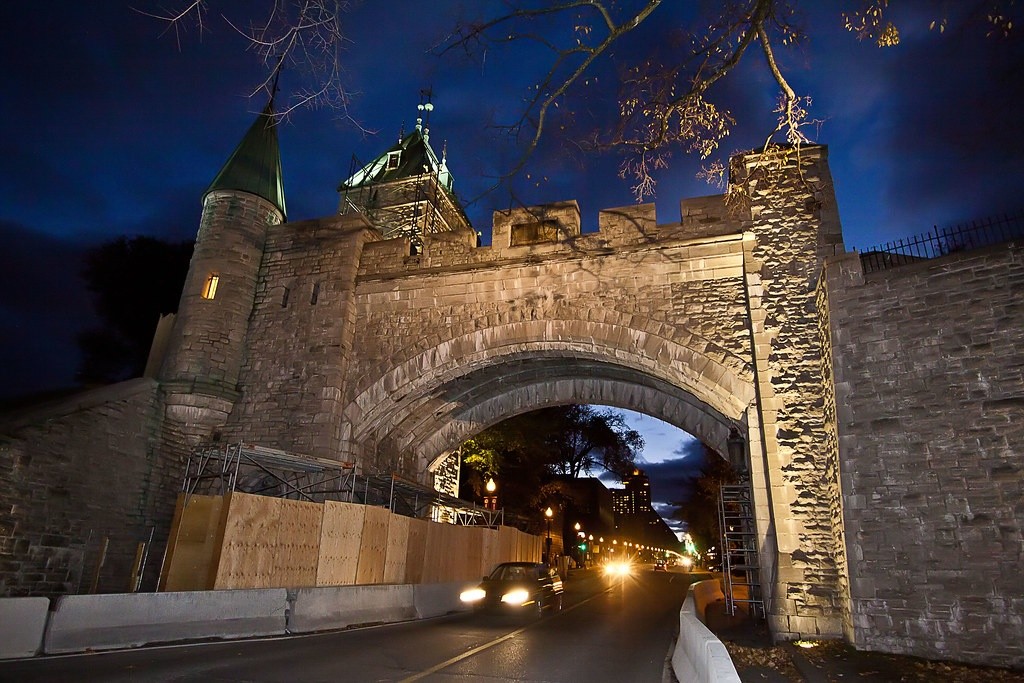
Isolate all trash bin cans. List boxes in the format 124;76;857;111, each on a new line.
555;555;570;580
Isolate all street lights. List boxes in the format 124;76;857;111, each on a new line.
486;477;496;510
599;523;684;556
546;505;554;564
575;522;594;570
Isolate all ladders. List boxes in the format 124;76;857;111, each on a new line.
717;480;766;619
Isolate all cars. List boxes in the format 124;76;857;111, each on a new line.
472;559;565;621
604;558;695;581
